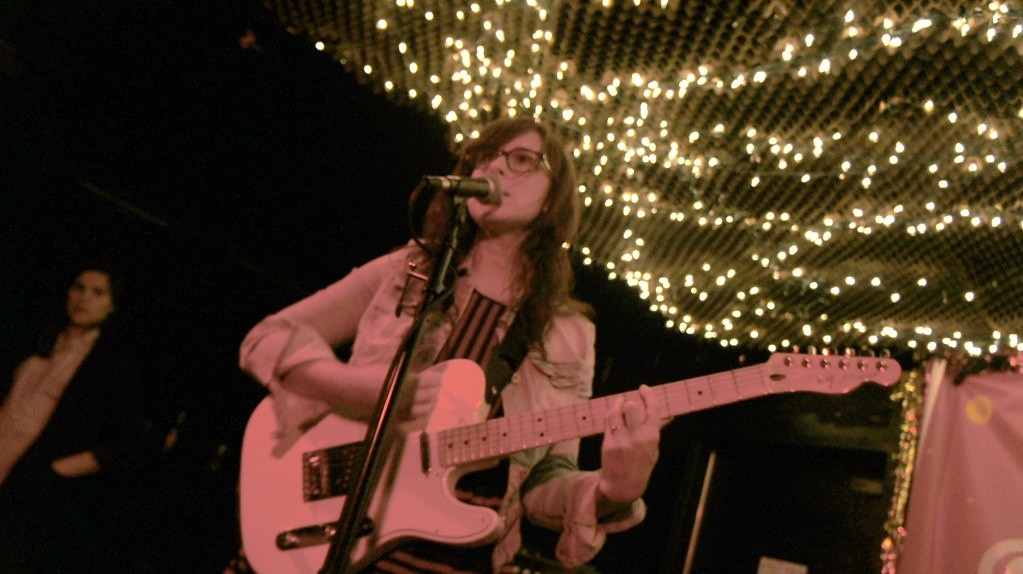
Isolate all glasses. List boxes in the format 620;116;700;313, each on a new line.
465;146;549;173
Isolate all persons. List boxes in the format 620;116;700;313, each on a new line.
223;119;675;574
0;260;144;519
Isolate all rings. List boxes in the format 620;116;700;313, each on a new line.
611;425;620;434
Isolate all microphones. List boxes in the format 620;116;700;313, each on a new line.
420;176;501;204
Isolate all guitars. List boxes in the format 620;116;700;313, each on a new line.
238;341;902;574
